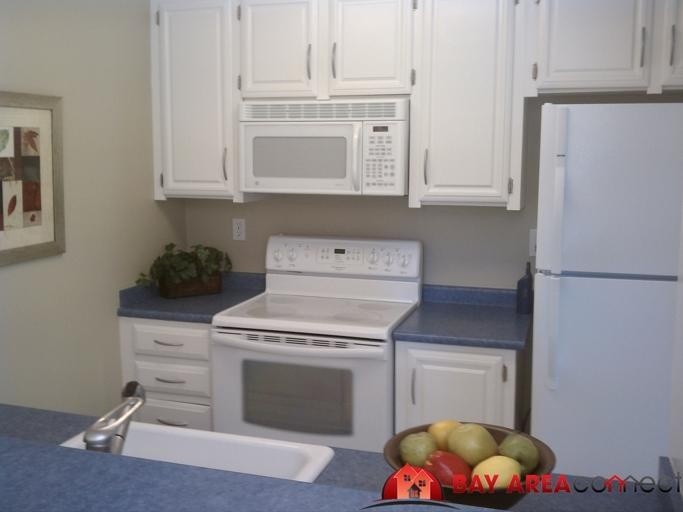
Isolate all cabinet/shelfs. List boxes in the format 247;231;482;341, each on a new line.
149;0;243;205
393;333;524;440
525;0;683;99
116;310;211;434
408;1;525;213
241;1;414;99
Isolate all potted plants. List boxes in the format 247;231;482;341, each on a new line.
134;241;232;298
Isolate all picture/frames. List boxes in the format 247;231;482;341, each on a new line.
0;91;66;269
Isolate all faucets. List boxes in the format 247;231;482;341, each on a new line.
82;381;147;455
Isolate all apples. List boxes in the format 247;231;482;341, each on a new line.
471;457;521;489
399;431;434;464
497;432;539;472
428;420;460;451
447;422;498;464
425;450;471;487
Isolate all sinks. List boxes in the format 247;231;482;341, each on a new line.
59;419;335;483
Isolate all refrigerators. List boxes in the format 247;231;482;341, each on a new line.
530;103;683;485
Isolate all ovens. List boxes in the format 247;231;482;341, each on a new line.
211;328;394;452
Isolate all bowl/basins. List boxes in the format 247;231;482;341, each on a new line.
384;422;556;510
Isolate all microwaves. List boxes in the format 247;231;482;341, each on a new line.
237;97;409;197
